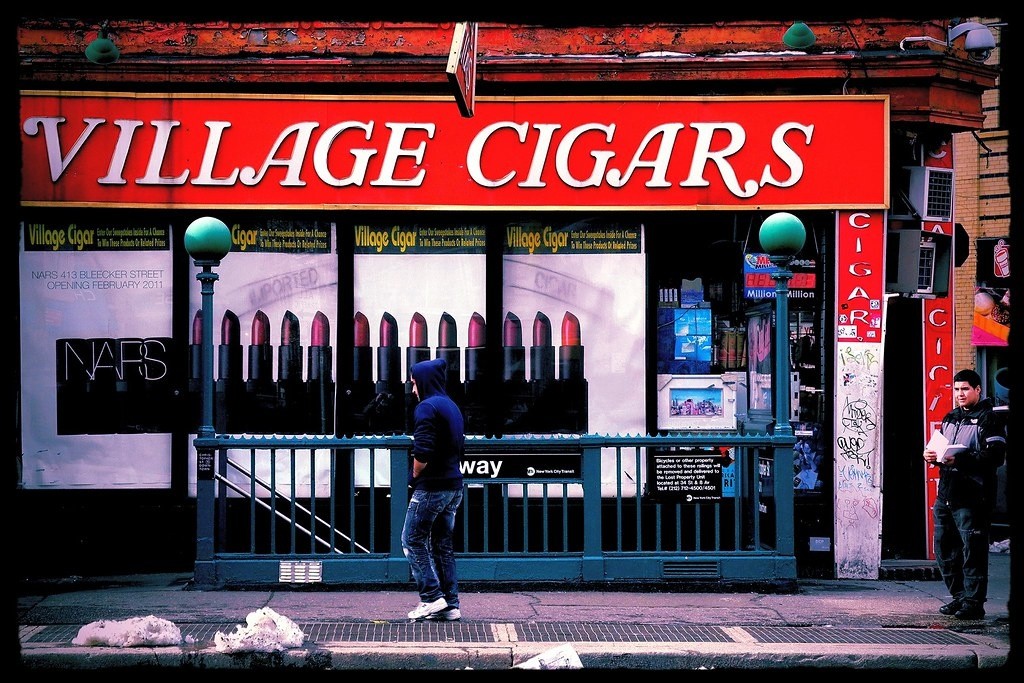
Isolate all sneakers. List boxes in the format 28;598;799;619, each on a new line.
408;597;448;618
424;608;461;621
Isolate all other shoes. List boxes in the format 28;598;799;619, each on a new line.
955;606;985;618
939;600;962;614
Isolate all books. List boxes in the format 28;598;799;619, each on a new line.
927;431;966;462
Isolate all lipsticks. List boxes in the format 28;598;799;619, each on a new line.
187;309;587;435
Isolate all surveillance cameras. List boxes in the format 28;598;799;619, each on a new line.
964;29;996;62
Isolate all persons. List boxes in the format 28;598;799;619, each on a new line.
400;359;465;622
923;369;1006;619
